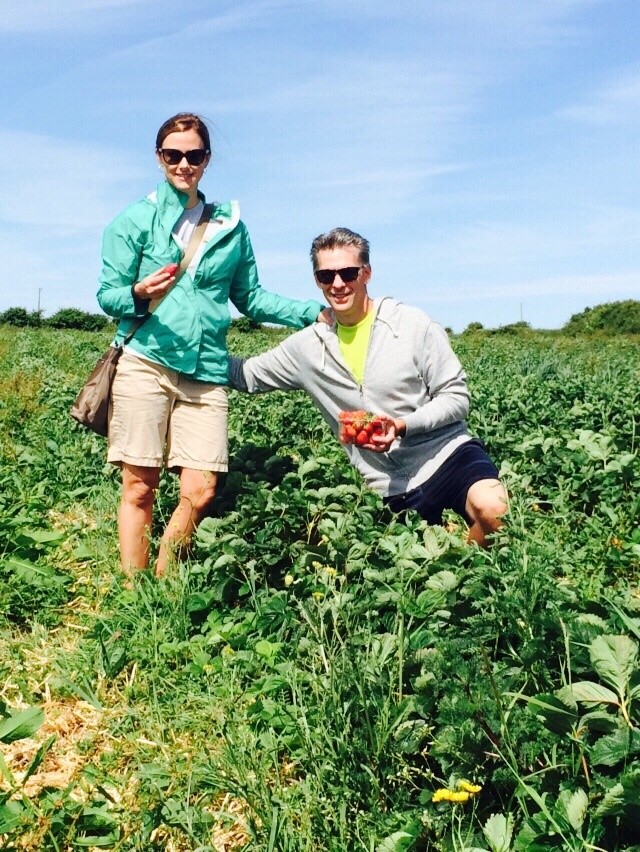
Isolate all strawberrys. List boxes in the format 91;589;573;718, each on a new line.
161;263;178;279
339;409;389;446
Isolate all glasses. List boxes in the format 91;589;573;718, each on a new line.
158;148;209;166
315;267;363;284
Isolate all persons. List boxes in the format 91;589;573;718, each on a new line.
227;229;508;548
95;112;333;592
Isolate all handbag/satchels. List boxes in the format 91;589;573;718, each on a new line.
68;347;121;437
